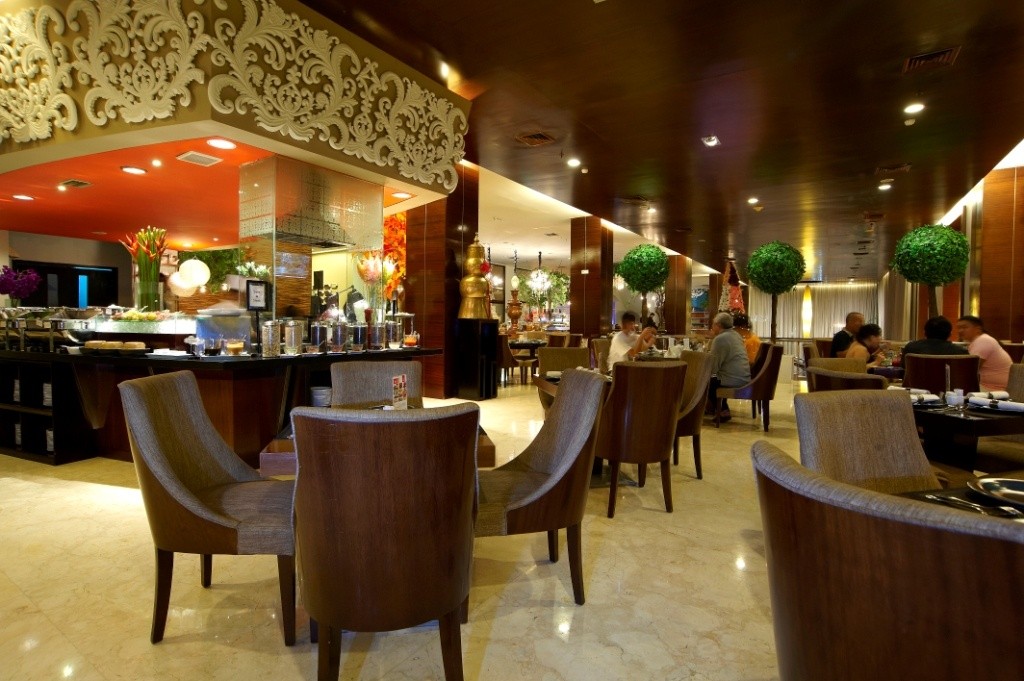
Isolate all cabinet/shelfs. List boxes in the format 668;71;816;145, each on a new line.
689;312;710;331
0;350;102;467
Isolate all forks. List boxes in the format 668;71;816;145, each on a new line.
936;491;1023;519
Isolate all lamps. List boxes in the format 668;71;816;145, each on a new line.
168;249;211;299
486;244;553;297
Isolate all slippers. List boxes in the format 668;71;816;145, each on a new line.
713;415;731;423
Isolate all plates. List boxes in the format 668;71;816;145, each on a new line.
966;476;1023;507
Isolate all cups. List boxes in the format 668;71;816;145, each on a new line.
206;336;244;355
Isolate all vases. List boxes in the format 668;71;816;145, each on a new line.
135;280;166;314
4;298;22;309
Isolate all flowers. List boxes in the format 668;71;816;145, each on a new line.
0;264;42;308
118;223;170;312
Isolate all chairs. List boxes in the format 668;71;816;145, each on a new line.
750;438;1024;681
748;343;774;418
285;402;480;681
794;390;942;500
714;345;784;432
325;359;426;408
499;334;536;387
667;350;713;480
115;369;300;646
592;361;688;521
537;347;596;414
476;366;611;609
547;332;585;347
804;335;1024;400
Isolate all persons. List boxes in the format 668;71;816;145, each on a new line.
955;315;1014;393
901;315;970;369
845;323;886;371
704;312;751;423
636;321;659;357
830;312;865;358
644;312;658;330
606;312;653;379
703;314;763;416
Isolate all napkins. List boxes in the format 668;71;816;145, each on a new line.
575;365;600;373
890;385;1024;411
546;370;564;379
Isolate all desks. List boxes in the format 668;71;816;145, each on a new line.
638;349;682;362
505;341;549;356
259;432;496;645
913;405;1024;474
877;479;1024;525
531;372;618;399
